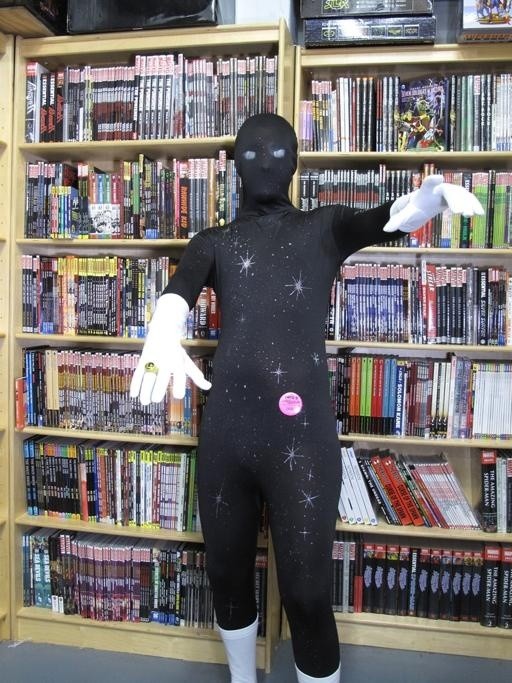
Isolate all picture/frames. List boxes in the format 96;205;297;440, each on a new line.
455;0;512;42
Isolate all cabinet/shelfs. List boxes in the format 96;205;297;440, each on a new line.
279;42;512;661
8;15;295;675
0;32;17;643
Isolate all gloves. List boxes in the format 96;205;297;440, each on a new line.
127;290;213;407
381;173;484;234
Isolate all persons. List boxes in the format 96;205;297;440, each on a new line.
127;112;484;678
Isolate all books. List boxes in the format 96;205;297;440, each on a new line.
327;353;512;441
13;345;213;438
23;253;222;342
23;435;268;535
299;162;512;248
19;528;266;636
336;442;512;533
24;54;279;143
332;533;512;629
323;261;512;345
297;73;512;153
24;149;242;240
